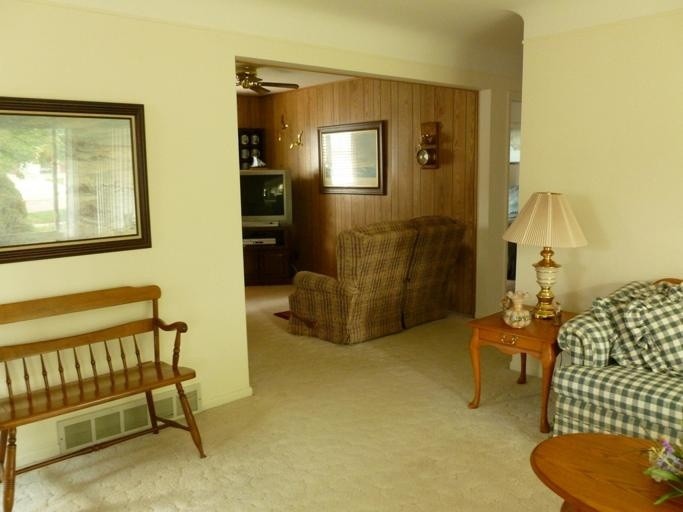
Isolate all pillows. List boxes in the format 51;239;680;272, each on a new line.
628;289;683;377
593;281;645;369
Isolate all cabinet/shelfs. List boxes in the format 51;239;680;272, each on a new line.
243;223;299;284
239;126;267;169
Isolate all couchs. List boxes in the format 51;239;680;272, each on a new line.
552;275;683;452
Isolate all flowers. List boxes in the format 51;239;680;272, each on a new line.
632;416;683;505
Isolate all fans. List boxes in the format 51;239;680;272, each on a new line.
236;66;299;95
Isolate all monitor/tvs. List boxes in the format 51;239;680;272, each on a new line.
240;169;292;232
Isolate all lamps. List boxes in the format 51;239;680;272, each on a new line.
237;73;262;89
501;191;588;318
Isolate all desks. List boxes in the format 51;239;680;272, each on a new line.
464;305;582;433
529;431;683;512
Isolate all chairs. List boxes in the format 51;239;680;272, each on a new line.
289;220;417;345
402;213;467;330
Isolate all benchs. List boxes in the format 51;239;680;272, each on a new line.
0;285;205;512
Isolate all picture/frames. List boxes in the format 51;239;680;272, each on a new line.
316;120;386;196
0;96;151;263
